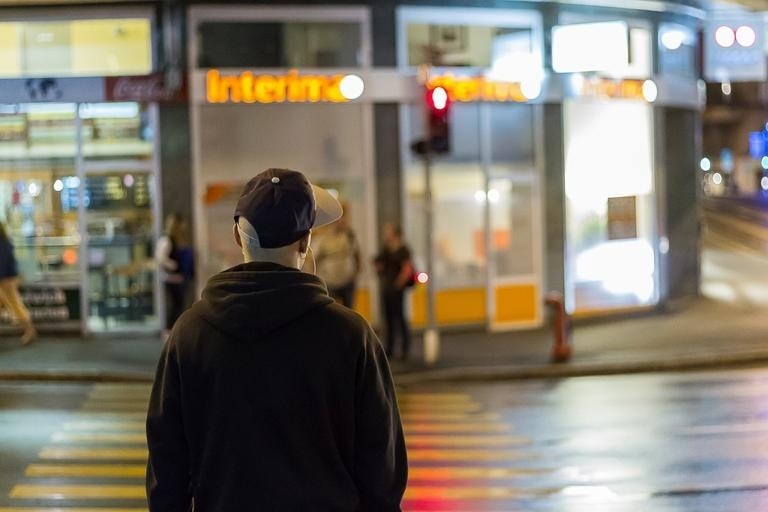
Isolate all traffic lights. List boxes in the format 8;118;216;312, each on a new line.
424;83;450;154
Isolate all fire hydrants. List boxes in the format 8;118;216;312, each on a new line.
539;291;573;364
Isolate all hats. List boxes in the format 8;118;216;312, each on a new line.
233;167;345;250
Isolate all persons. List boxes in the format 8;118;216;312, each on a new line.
144;165;408;510
152;210;196;344
370;218;415;364
1;222;38;347
310;200;362;311
